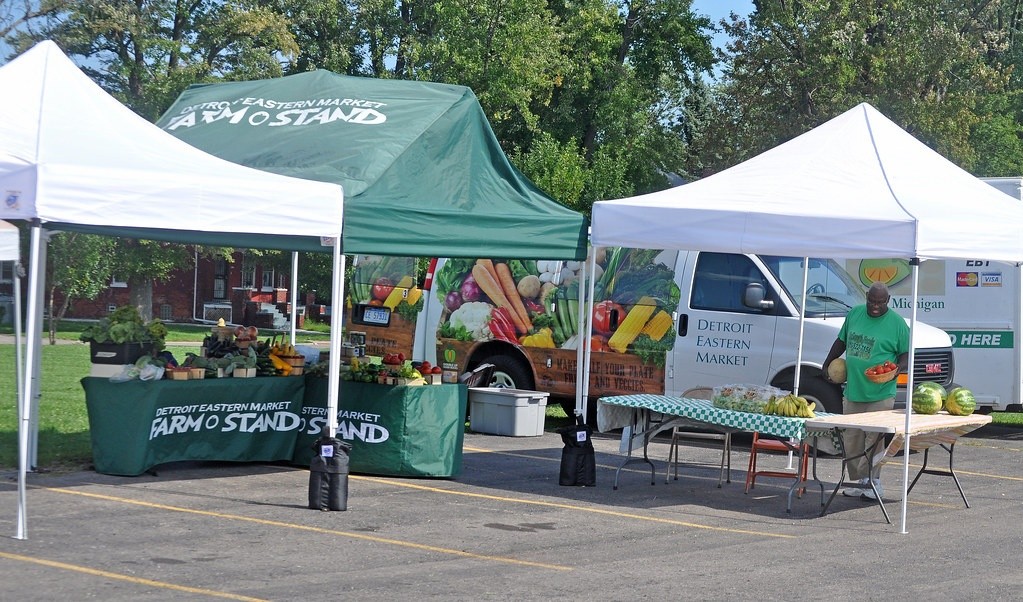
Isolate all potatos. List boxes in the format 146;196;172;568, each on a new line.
517;275;540;300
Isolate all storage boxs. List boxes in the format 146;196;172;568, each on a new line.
466;387;550;437
215;367;232;379
199;346;208;357
88;339;155;365
211;327;235;345
90;362;129;379
442;370;458;383
423;374;442;385
233;368;256;378
377;376;396;386
318;351;371;364
184;366;206;380
396;377;425;387
165;368;190;380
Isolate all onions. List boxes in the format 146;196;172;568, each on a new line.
446;273;480;311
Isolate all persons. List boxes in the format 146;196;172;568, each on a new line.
820;280;911;499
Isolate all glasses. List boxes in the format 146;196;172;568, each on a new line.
865;295;888;306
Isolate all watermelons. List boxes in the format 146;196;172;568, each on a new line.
911;381;976;415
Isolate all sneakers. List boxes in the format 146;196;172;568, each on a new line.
842;479;869;496
860;479;883;499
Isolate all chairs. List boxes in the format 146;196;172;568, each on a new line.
665;387;731;488
744;432;809;498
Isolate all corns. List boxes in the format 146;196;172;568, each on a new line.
382;275;421;313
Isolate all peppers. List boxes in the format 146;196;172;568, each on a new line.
339;362;386;382
485;305;521;347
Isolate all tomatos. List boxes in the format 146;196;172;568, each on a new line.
582;300;625;353
415;361;441;374
370;277;394;307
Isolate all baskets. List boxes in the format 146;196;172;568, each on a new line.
863;364;898;383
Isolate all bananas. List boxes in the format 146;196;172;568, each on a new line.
714;385;815;417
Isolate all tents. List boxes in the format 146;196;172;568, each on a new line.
0;220;26;435
31;66;591;475
580;101;1023;535
0;38;345;541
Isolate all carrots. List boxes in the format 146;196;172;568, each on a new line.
472;258;533;334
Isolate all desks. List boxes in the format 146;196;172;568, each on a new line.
599;394;842;514
805;408;993;524
81;375;306;478
293;373;468;478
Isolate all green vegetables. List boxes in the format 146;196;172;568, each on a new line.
79;305;167;350
346;256;389;304
396;257;472;342
594;246;681;370
